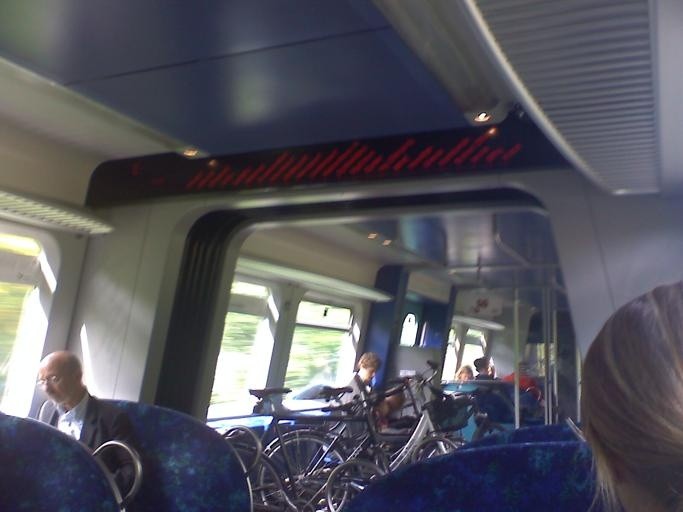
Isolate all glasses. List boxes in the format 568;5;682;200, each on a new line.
36;374;60;389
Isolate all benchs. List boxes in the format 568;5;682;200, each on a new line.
85;398;254;511
0;410;147;511
335;373;609;512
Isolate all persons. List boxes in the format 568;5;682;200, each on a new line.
346;352;381;438
455;366;473;381
504;362;536;396
35;351;144;512
474;356;497;379
579;279;683;512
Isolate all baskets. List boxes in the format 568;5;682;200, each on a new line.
422;396;468;433
